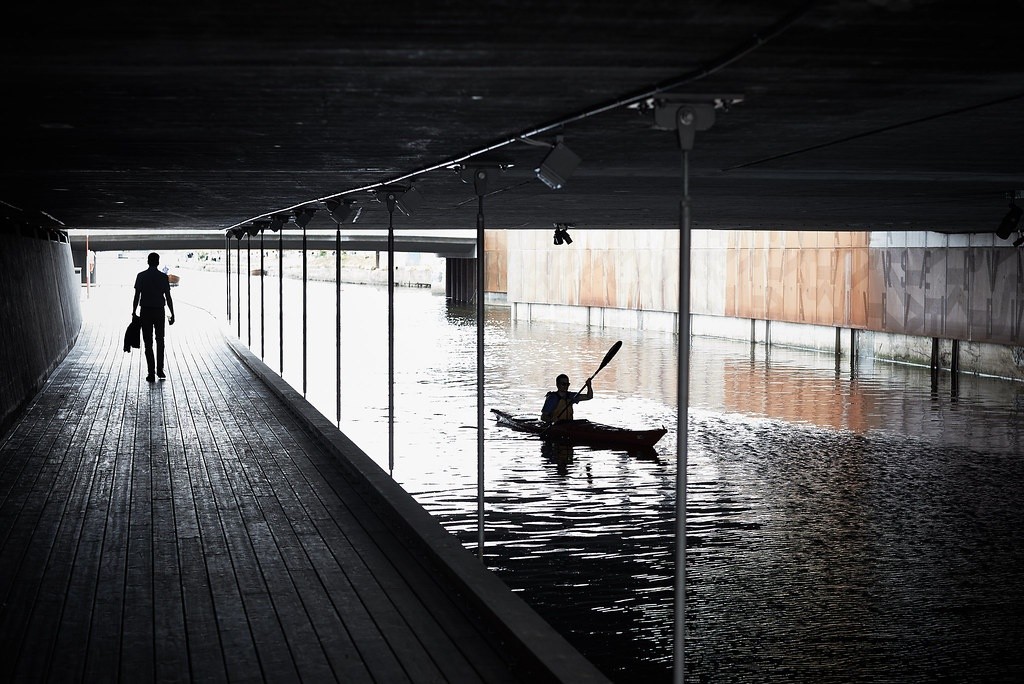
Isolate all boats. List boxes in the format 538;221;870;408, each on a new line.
166;274;180;286
251;269;267;275
492;405;667;450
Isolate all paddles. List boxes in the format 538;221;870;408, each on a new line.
539;339;623;435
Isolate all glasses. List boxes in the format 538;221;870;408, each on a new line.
559;382;570;386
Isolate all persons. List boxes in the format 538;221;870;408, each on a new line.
540;374;593;428
132;253;174;383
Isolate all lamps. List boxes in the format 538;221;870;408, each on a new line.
226;220;271;240
552;222;563;246
294;209;317;229
269;214;290;232
325;198;353;224
561;223;576;245
533;135;583;190
394;177;426;216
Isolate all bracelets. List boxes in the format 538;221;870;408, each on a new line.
132;313;136;315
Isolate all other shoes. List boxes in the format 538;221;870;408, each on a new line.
146;374;155;381
157;372;166;378
